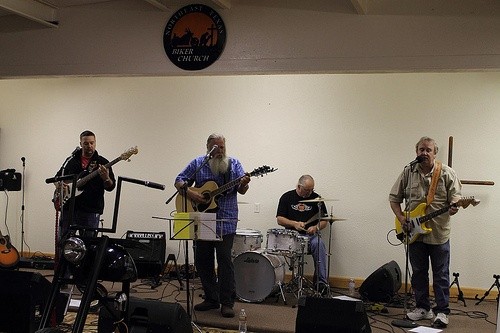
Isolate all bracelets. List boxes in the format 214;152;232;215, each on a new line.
316;224;319;231
104;178;111;182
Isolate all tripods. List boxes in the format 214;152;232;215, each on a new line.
283;203;334;309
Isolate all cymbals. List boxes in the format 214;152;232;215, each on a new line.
298;198;341;202
316;217;349;222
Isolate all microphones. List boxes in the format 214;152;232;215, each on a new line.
405;156;424;168
21;157;25;167
207;144;218;157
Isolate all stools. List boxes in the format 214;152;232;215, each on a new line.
289;253;311;292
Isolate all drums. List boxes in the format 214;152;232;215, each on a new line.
230;227;264;259
264;227;299;255
232;248;286;303
296;235;308;255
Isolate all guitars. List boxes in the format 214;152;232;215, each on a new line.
175;164;279;214
53;145;139;211
394;195;481;246
0;230;20;270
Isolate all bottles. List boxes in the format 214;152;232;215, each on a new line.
239;308;247;333
349;279;355;295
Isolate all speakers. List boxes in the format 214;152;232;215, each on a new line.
0;270;58;333
126;230;166;278
358;260;402;303
97;297;193;333
295;296;373;333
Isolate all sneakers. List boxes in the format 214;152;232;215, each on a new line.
433;312;449;327
405;308;433;321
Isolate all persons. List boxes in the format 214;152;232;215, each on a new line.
174;133;251;318
53;130;116;291
388;135;462;327
275;175;329;294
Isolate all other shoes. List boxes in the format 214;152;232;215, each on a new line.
194;300;220;311
221;304;234;318
